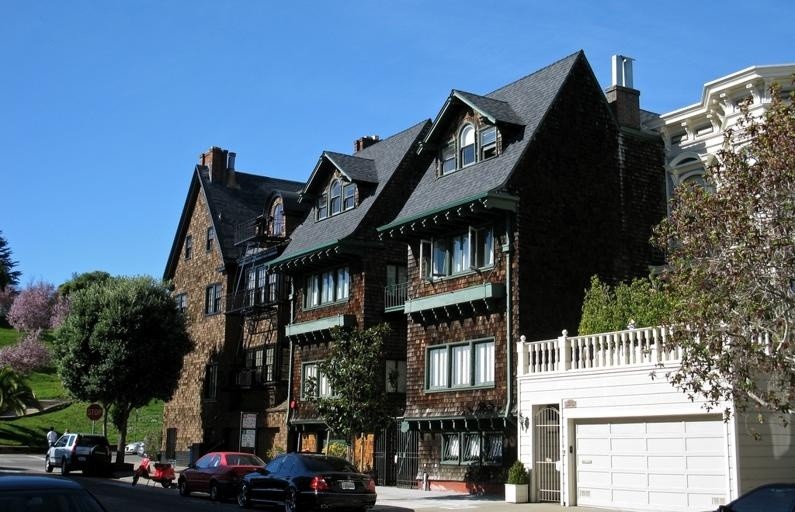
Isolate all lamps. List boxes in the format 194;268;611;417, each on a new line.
519;412;526;431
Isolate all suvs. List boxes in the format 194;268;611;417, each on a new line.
44;432;112;477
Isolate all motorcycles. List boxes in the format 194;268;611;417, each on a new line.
132;453;176;488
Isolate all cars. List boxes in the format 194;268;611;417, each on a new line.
235;452;377;512
0;472;108;512
125;440;161;458
178;452;266;501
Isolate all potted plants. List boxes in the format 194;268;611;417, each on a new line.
505;462;529;500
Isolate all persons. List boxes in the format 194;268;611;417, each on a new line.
63;428;70;435
46;426;57;448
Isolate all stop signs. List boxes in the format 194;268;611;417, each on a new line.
86;404;103;421
